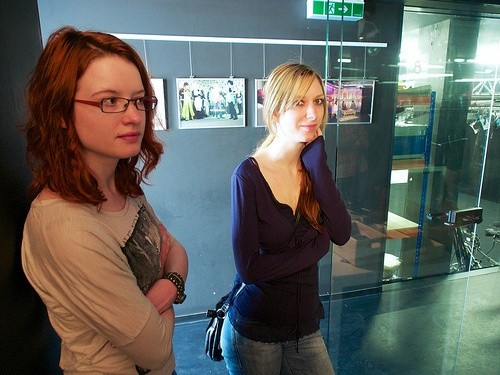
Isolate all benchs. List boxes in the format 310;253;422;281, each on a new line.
332;153;447;287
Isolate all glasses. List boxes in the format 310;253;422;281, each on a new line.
73;96;158;114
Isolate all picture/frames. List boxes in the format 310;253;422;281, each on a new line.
321;79;374;124
255;78;270;128
175;78;245;128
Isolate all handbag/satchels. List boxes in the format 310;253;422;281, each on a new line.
201;295;231;363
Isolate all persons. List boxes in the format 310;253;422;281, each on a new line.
178;80;243;120
21;25;189;375
219;61;352;375
332;100;356;118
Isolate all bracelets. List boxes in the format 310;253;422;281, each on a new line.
161;272;187;305
